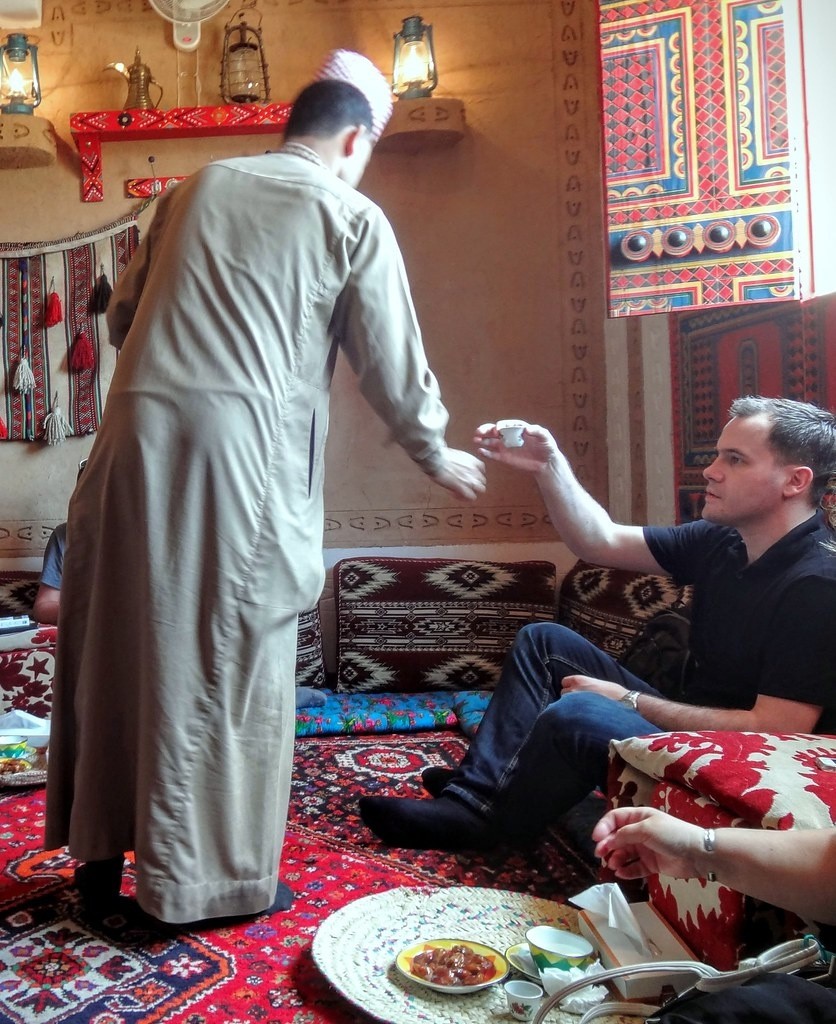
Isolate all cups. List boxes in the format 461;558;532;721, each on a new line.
504;980;543;1022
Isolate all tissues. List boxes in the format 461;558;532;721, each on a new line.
567;882;701;1004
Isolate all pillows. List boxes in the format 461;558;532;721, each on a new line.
295;597;328;691
333;557;558;693
555;558;696;664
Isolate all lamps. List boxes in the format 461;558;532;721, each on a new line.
0;32;42;115
217;6;271;104
392;16;438;101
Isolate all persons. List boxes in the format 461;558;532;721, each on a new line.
43;82;486;928
33;468;85;623
361;396;835;859
590;806;836;1024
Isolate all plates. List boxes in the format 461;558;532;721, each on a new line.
396;940;509;994
505;943;594;985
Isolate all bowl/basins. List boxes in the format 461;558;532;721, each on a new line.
525;926;593;973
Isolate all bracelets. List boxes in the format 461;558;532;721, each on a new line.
706;829;716;882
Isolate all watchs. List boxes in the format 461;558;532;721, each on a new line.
621;691;643;710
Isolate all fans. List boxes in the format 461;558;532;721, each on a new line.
149;0;230;53
